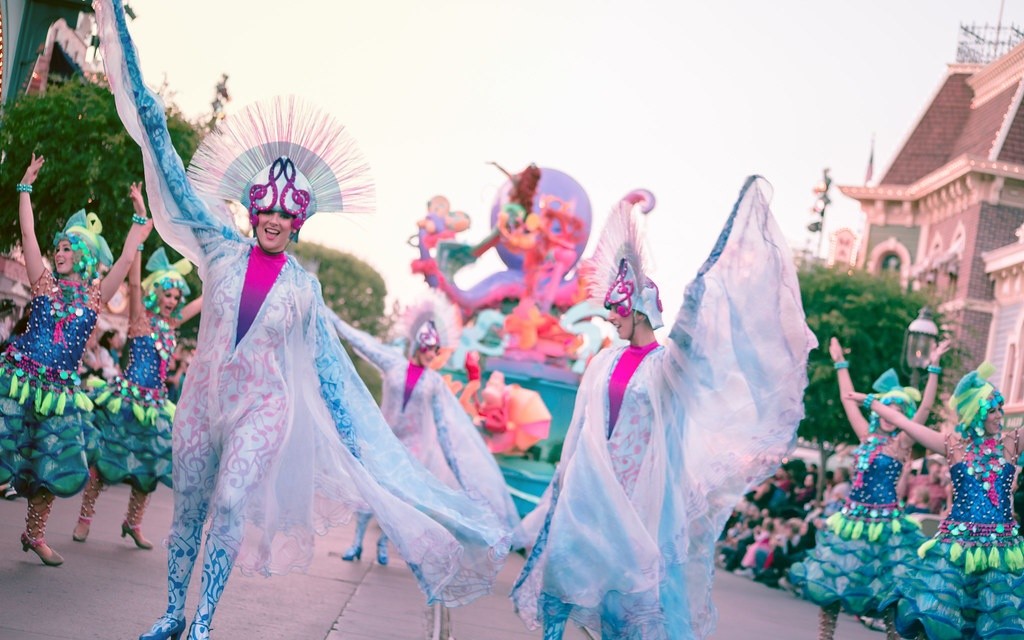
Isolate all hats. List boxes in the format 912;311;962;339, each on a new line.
949;361;1004;445
53;209;114;279
870;367;922;436
604;257;663;329
239;155;317;230
142;246;193;319
411;320;440;357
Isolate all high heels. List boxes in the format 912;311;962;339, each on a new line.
72;515;93;541
20;532;63;565
121;519;153;549
377;539;388;564
138;616;210;640
342;545;362;561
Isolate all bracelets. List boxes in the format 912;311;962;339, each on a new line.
863;393;874;408
834;360;850;368
138;243;144;250
131;214;148;225
927;365;942;375
17;184;33;193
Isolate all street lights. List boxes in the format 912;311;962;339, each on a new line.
901;306;938;387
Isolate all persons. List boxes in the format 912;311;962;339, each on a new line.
0;153;1024;640
91;1;362;640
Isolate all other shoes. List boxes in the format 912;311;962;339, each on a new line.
0;483;17;500
